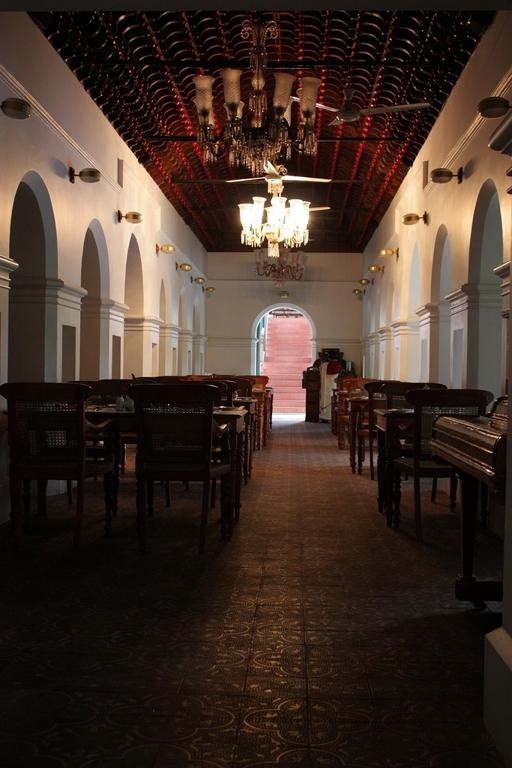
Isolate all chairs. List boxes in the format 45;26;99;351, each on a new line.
331;374;495;542
0;371;274;554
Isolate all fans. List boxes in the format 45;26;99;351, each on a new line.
263;193;334;213
221;160;333;185
284;74;430;127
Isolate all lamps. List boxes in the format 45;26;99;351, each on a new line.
428;166;464;184
156;243;216;296
280;290;290;298
475;94;512;120
189;12;324;175
251;247;308;286
117;209;144;225
1;97;31;120
401;210;430;226
66;164;101;184
351;247;399;295
231;192;314;257
